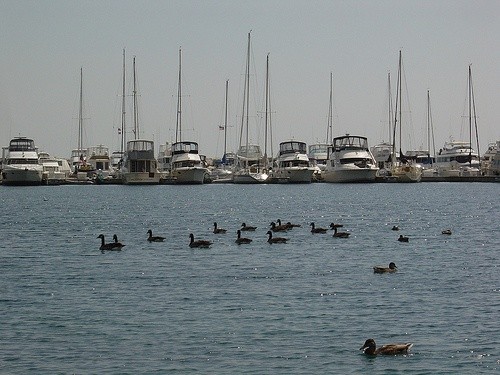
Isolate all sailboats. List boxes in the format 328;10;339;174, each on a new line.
37;30;500;184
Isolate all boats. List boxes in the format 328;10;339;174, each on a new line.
276;136;319;183
0;132;43;184
171;141;208;184
320;133;380;183
122;138;162;183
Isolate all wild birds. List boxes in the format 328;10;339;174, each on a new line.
330;225;351;238
310;221;328;233
358;338;411;358
269;219;300;231
398;234;409;243
391;226;399;230
373;262;397;274
235;229;254;244
214;221;228;233
241;222;257;232
147;229;167;243
95;234;125;251
442;229;451;235
188;232;214;247
266;231;287;243
330;222;343;228
